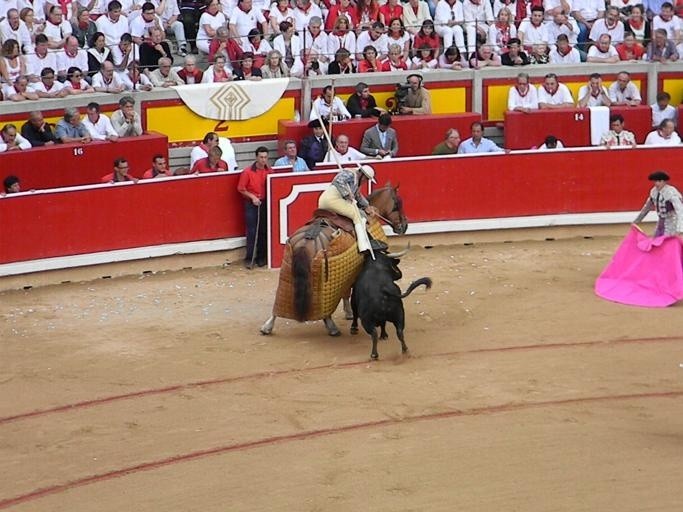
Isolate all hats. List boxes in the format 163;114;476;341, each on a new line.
353;160;375;185
305;118;328;128
648;171;670;180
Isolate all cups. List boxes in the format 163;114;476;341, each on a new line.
329;116;335;121
334;116;338;121
340;115;346;120
356;115;362;119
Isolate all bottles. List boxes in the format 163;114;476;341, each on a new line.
293;110;301;127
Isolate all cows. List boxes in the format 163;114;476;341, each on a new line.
350;241;433;362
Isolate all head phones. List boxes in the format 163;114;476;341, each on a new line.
406;74;424;89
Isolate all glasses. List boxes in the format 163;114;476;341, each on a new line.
117;166;129;169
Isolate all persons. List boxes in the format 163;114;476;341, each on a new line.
238;146;275;268
318;164;377;252
632;171;683;238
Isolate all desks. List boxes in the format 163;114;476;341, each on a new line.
501;101;653;149
276;112;484;164
0;130;171;193
677;103;682;140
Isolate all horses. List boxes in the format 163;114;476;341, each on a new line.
259;180;408;337
